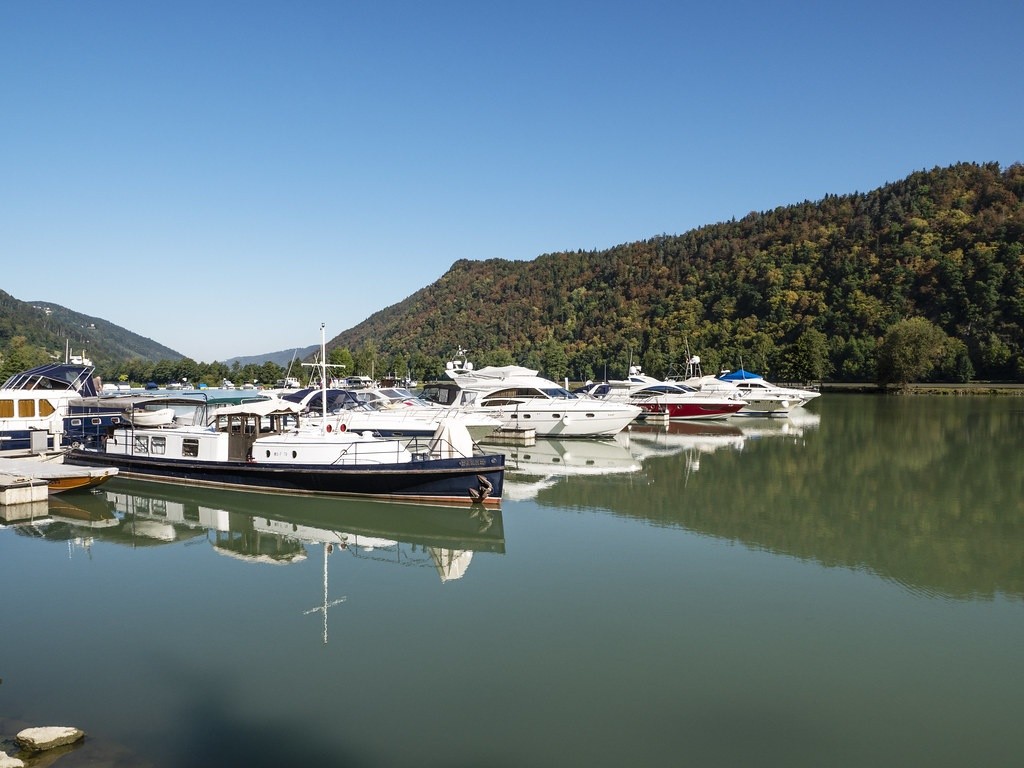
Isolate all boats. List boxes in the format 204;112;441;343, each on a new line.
46;488;121;530
110;469;509;647
621;419;750;481
731;407;805;441
475;435;648;480
0;455;121;493
0;324;822;516
791;407;821;431
